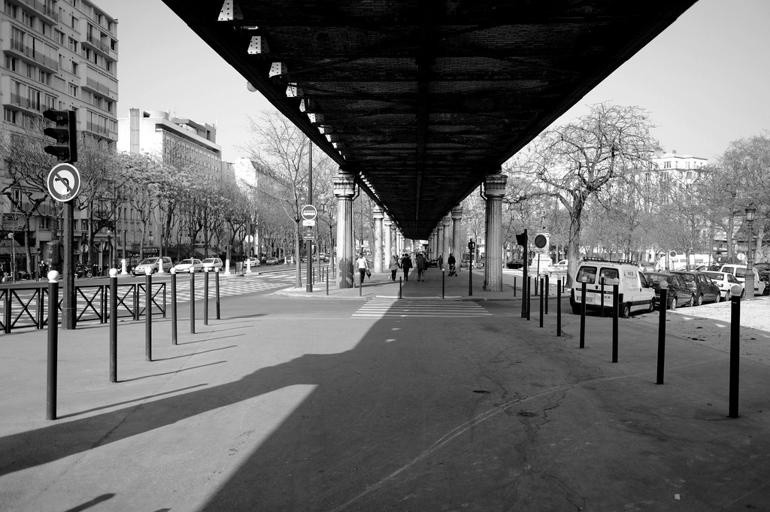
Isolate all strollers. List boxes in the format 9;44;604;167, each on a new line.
448;265;458;276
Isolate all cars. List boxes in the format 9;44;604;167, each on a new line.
670;271;721;305
702;270;746;301
302;252;336;263
201;258;223;271
266;257;279;265
555;259;568;265
76;264;94;277
244;257;261;267
643;271;695;311
174;259;203;274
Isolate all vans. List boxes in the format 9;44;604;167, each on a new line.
719;264;770;297
570;258;656;318
134;255;174;275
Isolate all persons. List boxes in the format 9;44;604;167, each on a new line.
357;253;368;283
448;263;455;275
448;254;455;268
438;255;443;270
390;249;430;282
38;259;47;283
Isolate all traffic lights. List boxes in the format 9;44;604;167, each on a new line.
516;232;528;246
42;107;77;163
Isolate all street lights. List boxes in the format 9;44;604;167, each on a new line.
745;203;757;300
